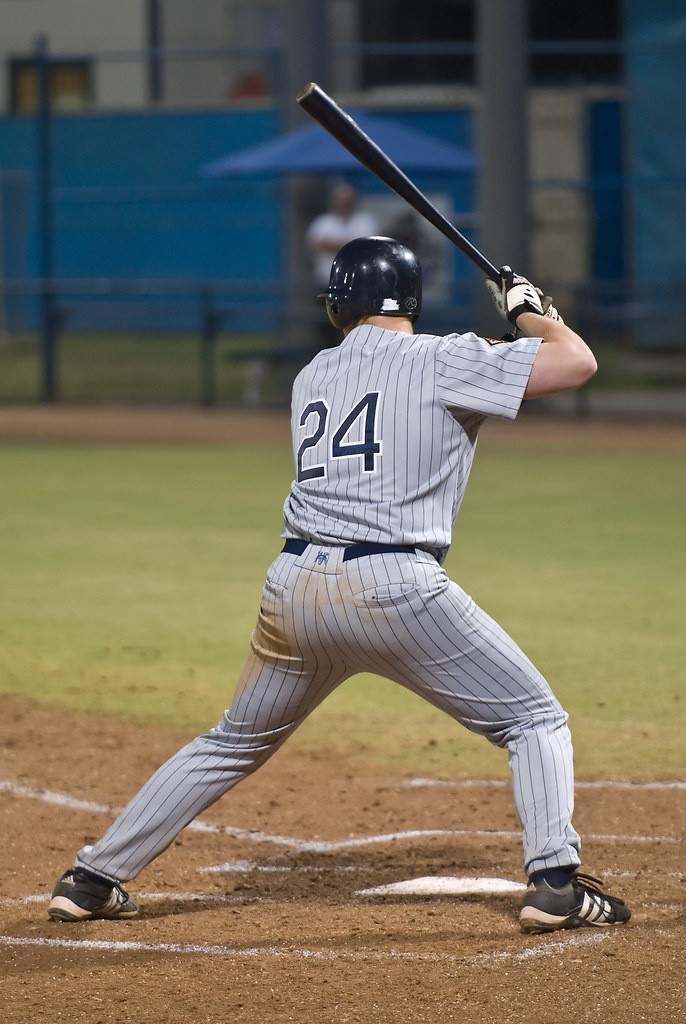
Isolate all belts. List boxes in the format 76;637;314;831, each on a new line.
281;538;416;563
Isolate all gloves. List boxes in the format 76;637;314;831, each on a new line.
485;266;544;333
513;288;565;338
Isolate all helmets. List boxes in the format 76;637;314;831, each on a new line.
317;236;423;331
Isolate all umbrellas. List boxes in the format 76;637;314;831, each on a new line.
199;93;478;186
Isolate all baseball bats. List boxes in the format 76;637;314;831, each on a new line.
294;80;501;282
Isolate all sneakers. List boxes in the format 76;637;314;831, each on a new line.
47;869;140;923
519;871;632;932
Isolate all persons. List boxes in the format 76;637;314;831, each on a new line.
47;236;633;932
305;182;379;345
